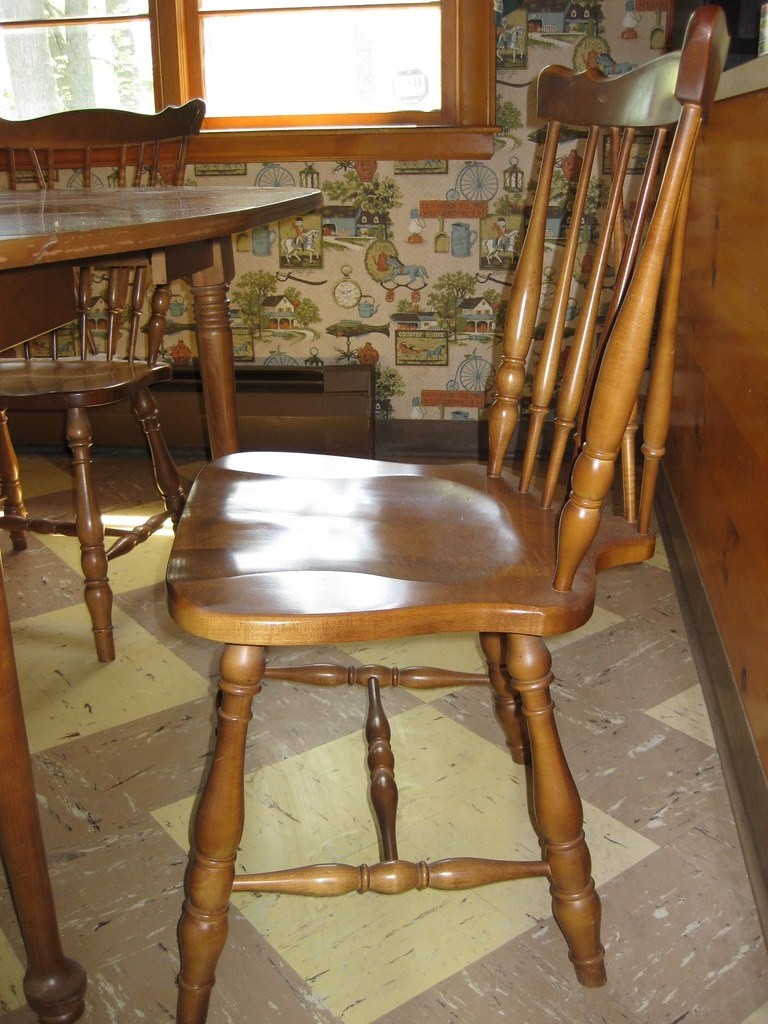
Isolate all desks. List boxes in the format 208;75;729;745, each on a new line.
0;186;325;1024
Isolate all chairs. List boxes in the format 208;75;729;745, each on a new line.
0;98;207;663
165;5;734;1023
